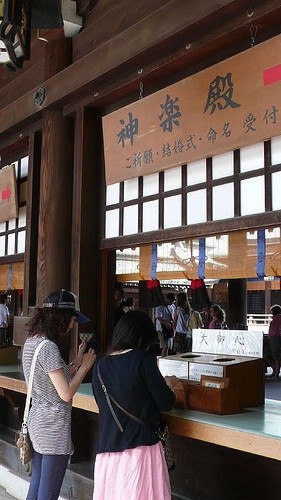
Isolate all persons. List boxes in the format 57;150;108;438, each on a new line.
123;296;135;314
113;281;125;327
264;304;281;381
208;304;227;329
171;292;193;355
21;289;98;500
200;301;214;329
0;294;12;345
190;311;204;331
153;293;175;357
90;309;182;500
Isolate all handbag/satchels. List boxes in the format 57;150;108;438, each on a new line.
167;328;174;338
154;421;176;472
16;425;33;465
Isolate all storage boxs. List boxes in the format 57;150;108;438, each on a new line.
157;329;265;415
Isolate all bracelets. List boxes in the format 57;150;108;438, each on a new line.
70;361;79;370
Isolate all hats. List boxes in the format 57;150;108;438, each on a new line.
167;293;177;302
43;289;92;324
